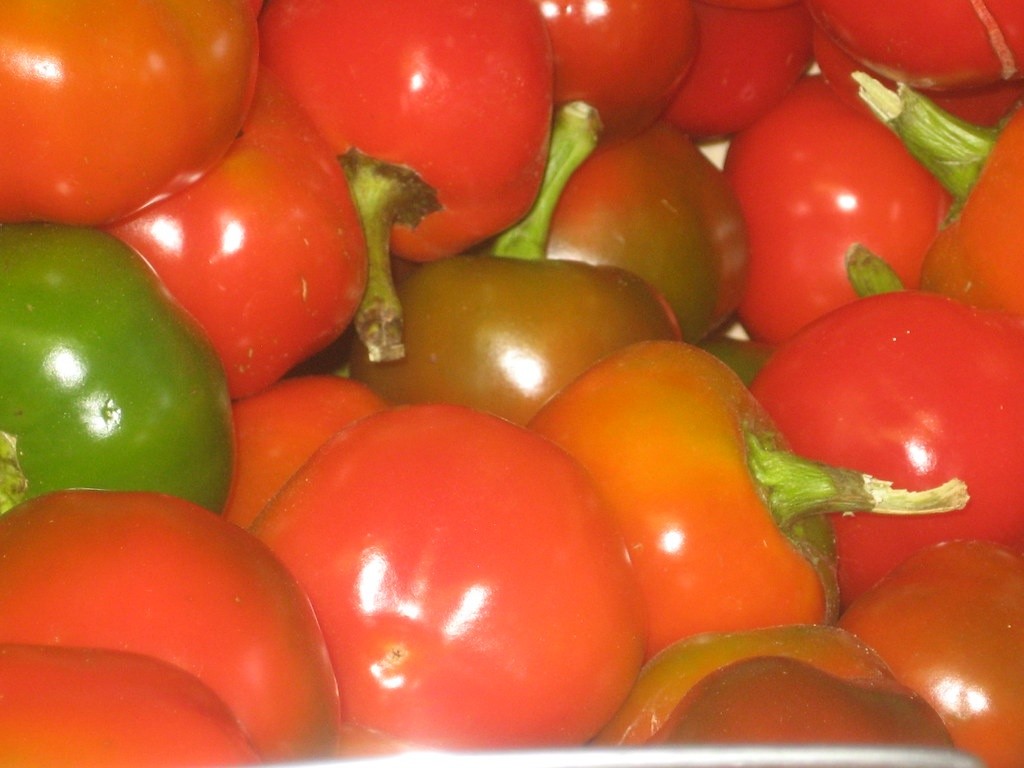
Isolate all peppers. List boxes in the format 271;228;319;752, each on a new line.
0;0;1024;768
